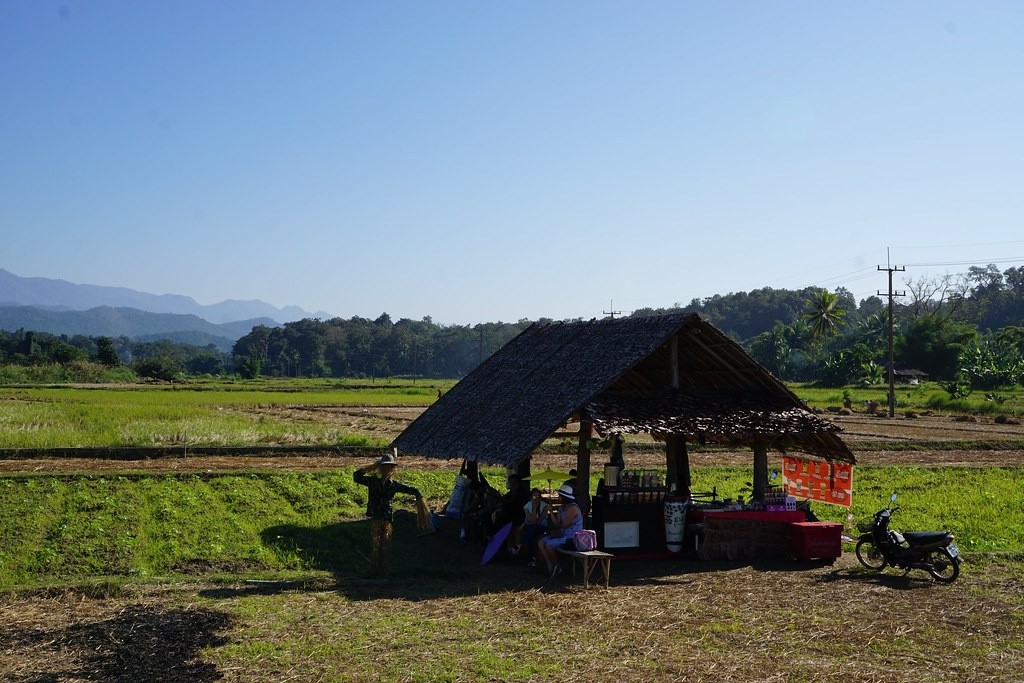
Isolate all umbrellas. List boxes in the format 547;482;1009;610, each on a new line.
522;465;577;505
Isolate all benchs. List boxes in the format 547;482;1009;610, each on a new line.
557;547;615;588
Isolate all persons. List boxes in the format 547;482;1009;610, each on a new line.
510;488;550;567
486;474;526;551
537;484;583;580
563;469;591;518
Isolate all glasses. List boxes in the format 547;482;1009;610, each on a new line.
532;495;540;499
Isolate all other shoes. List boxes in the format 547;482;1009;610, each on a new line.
512;543;520;556
528;556;537;567
545;565;563;581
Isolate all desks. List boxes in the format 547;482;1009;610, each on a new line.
598;486;805;551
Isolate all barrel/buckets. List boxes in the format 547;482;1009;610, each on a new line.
604;465;620;486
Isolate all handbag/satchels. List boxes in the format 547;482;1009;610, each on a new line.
560;529;597;551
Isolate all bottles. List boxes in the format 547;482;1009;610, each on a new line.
763;488;785;505
622;469;664;487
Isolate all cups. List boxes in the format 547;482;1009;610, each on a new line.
608;492;665;503
808;482;814;495
797;483;802;492
820;483;826;496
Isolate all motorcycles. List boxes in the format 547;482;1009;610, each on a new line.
856;494;964;583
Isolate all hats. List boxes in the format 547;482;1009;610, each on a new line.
557;485;575;499
378;454;398;466
